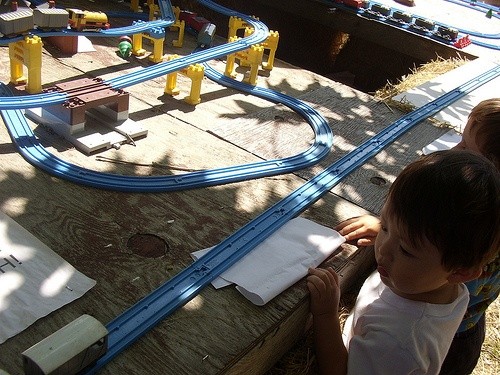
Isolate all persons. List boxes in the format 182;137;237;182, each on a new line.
308;150;500;375
447;97;500;375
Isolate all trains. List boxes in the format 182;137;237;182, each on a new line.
0;8;110;38
180;9;218;49
